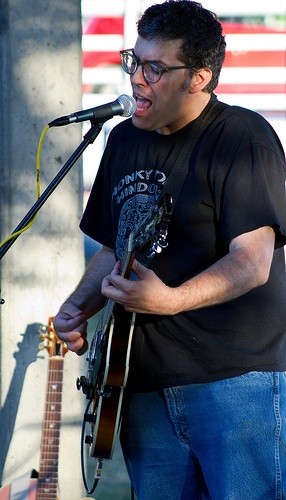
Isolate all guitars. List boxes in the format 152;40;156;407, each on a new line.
35;316;68;500
76;192;174;459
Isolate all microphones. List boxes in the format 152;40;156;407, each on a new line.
48;94;137;127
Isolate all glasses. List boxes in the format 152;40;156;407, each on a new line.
119;48;193;83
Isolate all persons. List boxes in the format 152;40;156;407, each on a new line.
53;0;286;500
